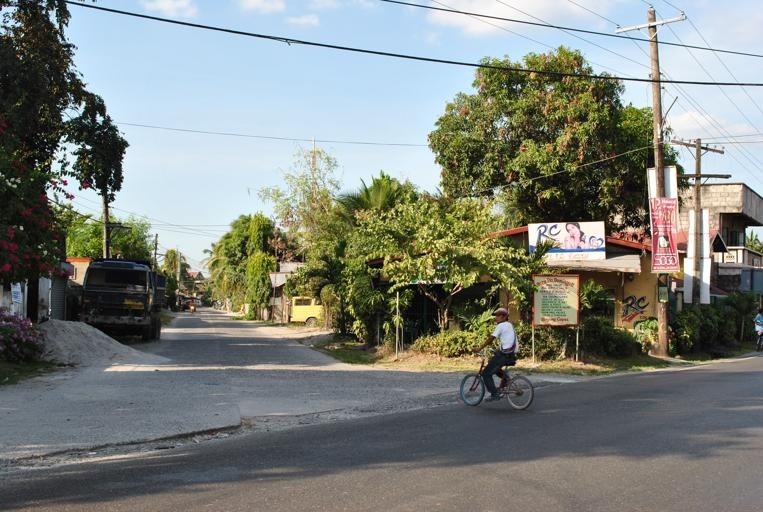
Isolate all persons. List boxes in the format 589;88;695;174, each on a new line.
751;308;762;345
470;307;520;402
562;223;588;250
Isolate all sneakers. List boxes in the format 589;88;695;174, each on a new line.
498;375;512;388
484;393;500;402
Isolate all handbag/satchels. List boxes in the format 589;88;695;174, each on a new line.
497;349;516;366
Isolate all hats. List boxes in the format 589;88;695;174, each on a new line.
494;308;509;317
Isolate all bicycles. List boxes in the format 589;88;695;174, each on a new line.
460;347;534;410
752;319;763;351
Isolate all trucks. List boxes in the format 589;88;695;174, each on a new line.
80;258;167;341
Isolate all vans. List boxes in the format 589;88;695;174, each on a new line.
290;296;324;326
